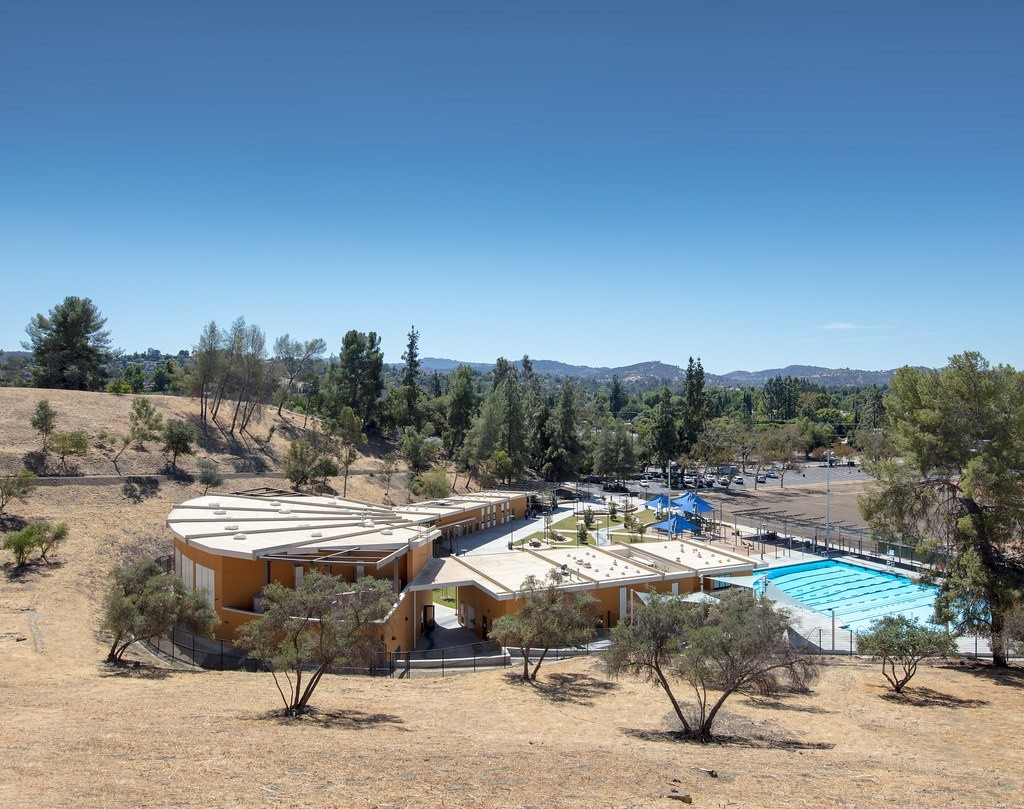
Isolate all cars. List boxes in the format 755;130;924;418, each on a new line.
766;471;778;478
718;478;729;485
684;471;716;488
757;476;766;484
638;479;651;488
731;476;744;485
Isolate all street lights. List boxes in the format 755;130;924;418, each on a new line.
509;514;516;543
937;483;957;572
542;510;549;545
667;458;678;542
762;523;767;554
454;525;462;556
823;449;838;551
574;496;581;548
595;518;600;546
